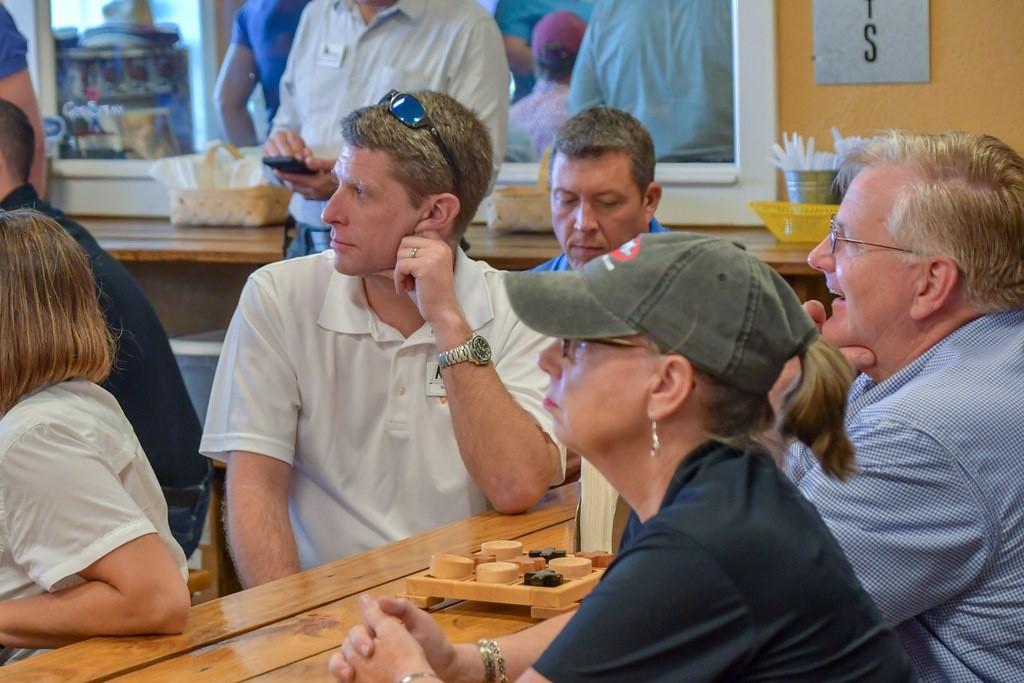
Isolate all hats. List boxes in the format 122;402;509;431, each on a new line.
502;232;816;396
533;10;586;62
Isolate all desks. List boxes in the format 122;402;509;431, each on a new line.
0;477;584;683
67;214;824;276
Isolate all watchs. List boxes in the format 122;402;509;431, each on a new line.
437;333;492;369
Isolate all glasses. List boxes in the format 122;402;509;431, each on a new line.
379;88;460;195
829;213;930;258
561;335;640;358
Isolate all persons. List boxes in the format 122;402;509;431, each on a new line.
0;97;215;560
329;232;923;683
198;90;568;588
215;0;735;166
273;0;515;253
773;130;1022;682
0;1;46;198
0;210;191;646
521;105;667;281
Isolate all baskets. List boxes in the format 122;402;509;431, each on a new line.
169;143;290;226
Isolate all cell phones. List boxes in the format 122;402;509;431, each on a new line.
262;156;317;175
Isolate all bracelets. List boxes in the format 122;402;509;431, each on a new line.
400;672;439;683
474;638;508;683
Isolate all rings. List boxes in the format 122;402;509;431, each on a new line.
412;248;416;257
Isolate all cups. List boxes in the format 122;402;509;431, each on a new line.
785;170;841;205
